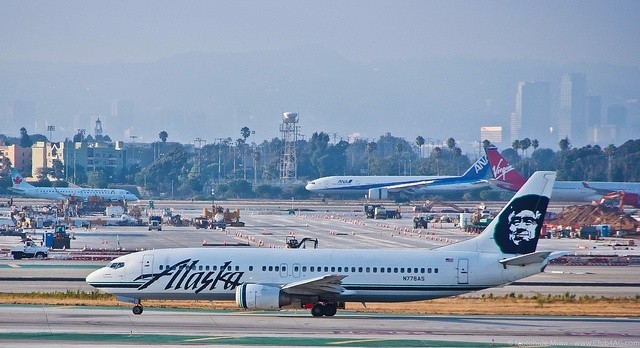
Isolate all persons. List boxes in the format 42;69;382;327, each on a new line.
508;206;541;251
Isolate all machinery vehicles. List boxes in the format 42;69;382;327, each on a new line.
52;224;71;249
364;203;403;227
201;202;246;232
411;212;430;227
283;232;322;248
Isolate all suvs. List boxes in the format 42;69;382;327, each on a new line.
148;214;163;231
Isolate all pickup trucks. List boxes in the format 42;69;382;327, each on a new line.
8;240;50;258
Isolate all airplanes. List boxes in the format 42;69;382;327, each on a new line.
484;141;639;207
304;139;497;205
86;168;570;317
6;167;139;205
